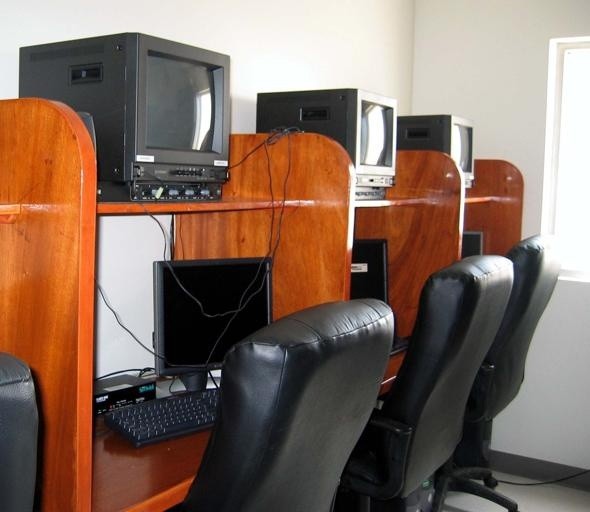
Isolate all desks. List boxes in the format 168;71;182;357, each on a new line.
0;97;525;512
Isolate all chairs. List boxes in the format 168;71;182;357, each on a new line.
431;234;561;512
339;255;513;512
178;298;394;511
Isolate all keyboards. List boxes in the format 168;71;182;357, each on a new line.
389;335;410;356
103;384;221;447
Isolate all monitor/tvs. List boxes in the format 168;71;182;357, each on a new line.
153;254;274;391
256;88;397;202
397;114;476;189
349;238;389;304
18;31;230;203
462;231;483;259
